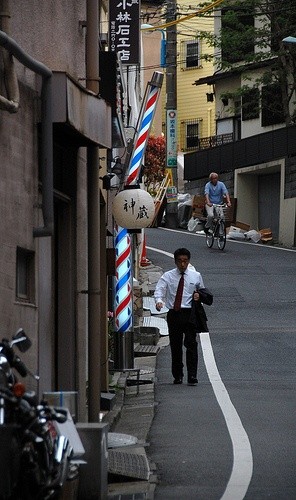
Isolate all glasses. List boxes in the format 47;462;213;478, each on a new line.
175;260;189;264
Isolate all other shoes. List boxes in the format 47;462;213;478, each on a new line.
174;377;182;384
204;227;209;234
188;376;199;386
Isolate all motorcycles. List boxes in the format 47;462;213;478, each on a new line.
0;328;86;500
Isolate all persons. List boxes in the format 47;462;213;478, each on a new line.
203;172;231;242
154;248;206;385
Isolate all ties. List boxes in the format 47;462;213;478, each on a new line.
174;273;184;312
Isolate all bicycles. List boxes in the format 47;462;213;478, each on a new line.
206;203;231;250
